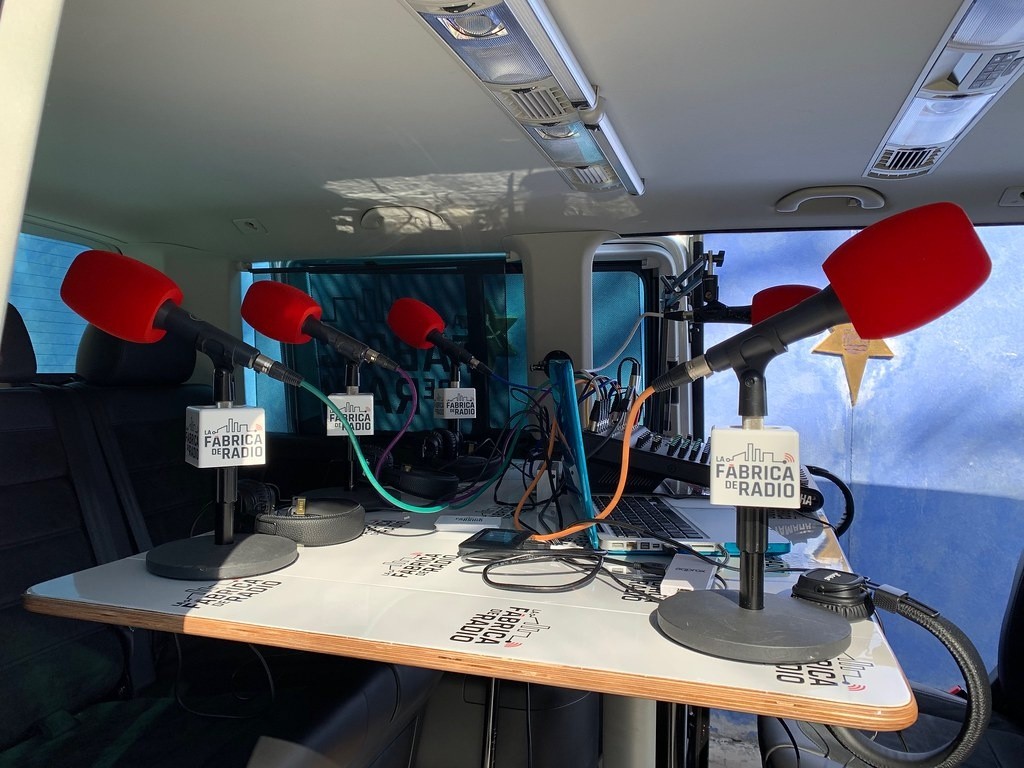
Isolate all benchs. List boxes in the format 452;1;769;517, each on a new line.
0;302;442;768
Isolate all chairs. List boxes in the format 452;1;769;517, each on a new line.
756;551;1024;768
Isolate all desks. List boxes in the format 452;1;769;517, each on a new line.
25;458;918;768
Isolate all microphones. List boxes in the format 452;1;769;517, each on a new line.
650;202;993;394
60;250;304;388
241;281;399;374
665;284;824;326
388;298;495;379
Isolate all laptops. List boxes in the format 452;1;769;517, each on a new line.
550;359;792;558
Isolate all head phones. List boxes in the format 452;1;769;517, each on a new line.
794;568;992;768
349;443;459;500
801;463;854;539
418;427;503;482
233;480;365;547
